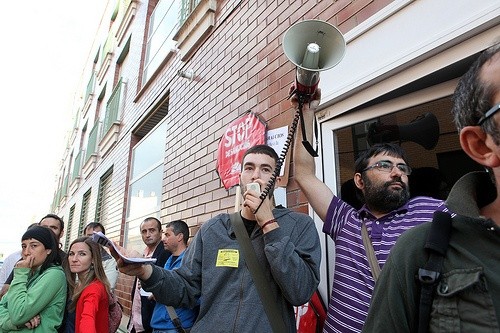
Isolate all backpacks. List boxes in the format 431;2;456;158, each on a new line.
108;290;123;333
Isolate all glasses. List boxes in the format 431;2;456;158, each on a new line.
360;161;412;175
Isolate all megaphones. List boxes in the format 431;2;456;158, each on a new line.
367;113;440;149
282;19;346;103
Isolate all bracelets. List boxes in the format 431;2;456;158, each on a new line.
261;217;278;230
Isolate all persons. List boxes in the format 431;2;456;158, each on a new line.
361;44;500;333
0;214;119;333
138;220;197;333
290;81;447;333
126;217;172;333
115;143;322;333
